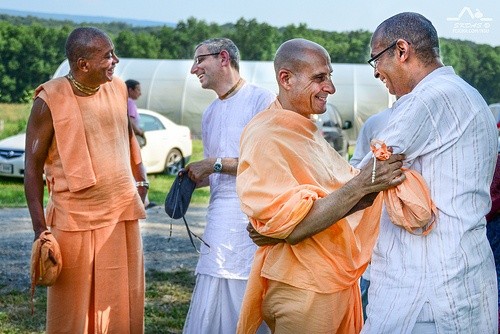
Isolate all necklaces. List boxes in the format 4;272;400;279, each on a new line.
67;71;99;96
219;77;243;100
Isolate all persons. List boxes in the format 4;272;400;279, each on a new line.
246;12;498;334
236;38;406;334
178;38;277;334
126;80;155;209
24;26;150;334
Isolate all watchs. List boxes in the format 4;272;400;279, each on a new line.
213;158;223;173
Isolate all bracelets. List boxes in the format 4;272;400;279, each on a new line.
136;181;149;188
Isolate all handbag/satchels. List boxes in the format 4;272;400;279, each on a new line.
30;230;62;288
371;139;439;236
165;169;196;220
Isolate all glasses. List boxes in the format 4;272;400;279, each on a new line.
367;39;411;69
193;52;220;64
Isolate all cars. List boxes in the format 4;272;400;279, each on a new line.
0;108;194;188
317;103;353;160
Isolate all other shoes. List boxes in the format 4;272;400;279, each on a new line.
144;201;156;211
